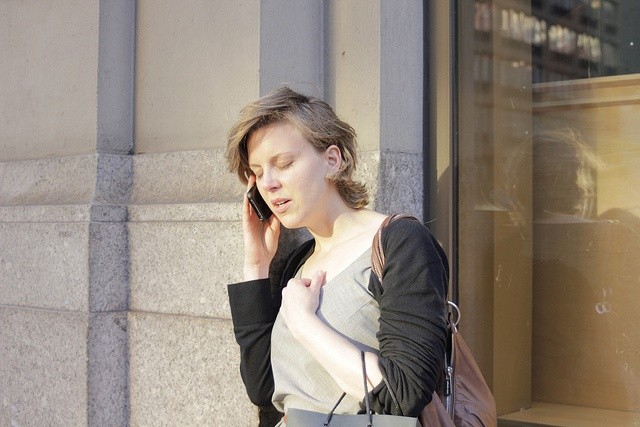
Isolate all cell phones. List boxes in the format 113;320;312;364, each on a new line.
247;185;273;222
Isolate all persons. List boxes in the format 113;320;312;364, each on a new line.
227;88;449;426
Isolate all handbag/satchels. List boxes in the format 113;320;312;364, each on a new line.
371;214;497;427
